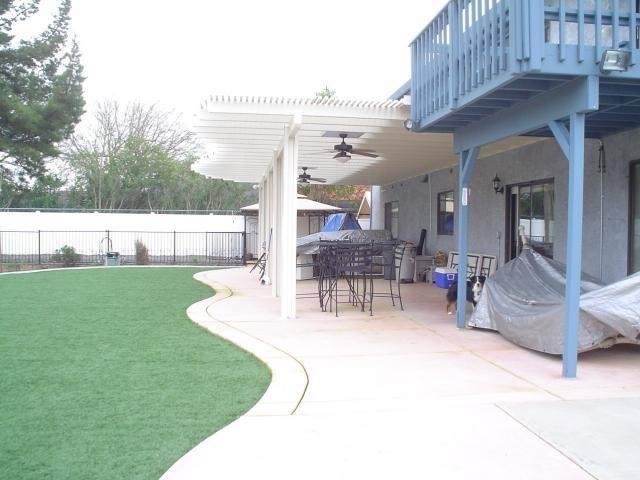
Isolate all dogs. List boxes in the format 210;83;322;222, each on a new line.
447;276;486;315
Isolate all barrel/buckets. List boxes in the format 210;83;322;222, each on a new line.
382;241;403;280
395;246;414;282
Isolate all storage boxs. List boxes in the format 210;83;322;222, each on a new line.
434;267;458;288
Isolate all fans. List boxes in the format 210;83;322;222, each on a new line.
297;167;328;183
303;134;379;158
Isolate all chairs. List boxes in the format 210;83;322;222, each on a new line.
295;235;407;317
249;252;266;280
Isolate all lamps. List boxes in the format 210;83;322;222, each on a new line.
492;172;504;194
600;50;632;74
403;118;415;130
300;179;310;186
334;153;351;163
